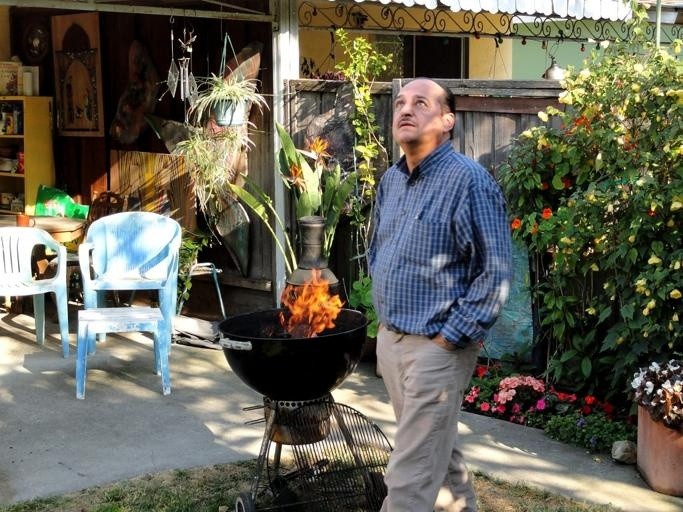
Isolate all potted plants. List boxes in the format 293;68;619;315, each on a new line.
185;83;276;131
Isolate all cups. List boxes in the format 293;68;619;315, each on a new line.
14;211;34;227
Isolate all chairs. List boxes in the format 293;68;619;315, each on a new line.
0;224;72;359
76;209;183;402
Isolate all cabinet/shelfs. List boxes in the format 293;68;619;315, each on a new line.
0;96;57;217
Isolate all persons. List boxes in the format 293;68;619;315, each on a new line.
362;75;515;512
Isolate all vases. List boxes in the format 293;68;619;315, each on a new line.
634;404;683;498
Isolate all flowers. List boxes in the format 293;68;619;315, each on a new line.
631;357;682;436
